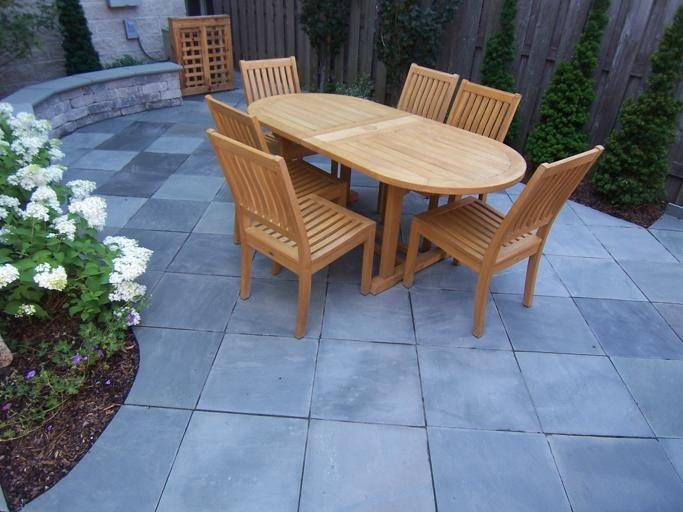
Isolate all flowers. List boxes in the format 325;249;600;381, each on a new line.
0;101;155;340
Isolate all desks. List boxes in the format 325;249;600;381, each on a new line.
245;91;527;297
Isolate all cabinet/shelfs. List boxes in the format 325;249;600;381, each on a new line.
167;14;235;97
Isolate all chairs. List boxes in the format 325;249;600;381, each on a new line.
403;145;605;338
237;55;341;179
419;78;524;265
205;128;377;340
376;64;461;214
203;92;346;273
